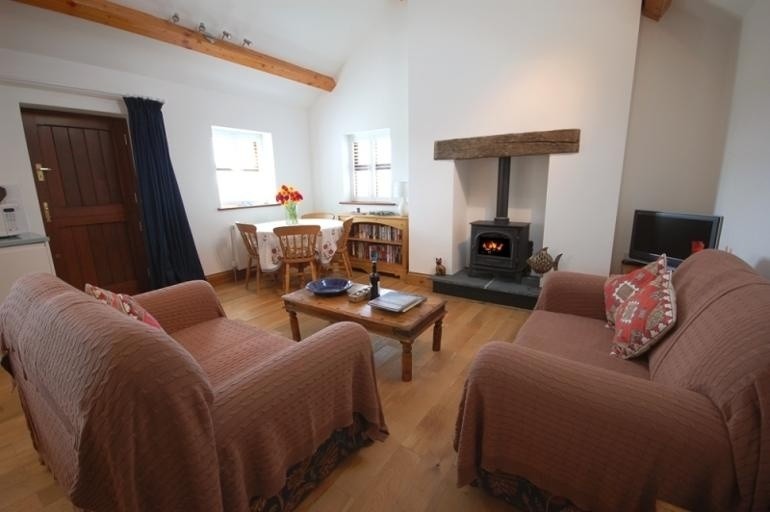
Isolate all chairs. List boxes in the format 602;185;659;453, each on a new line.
233;222;262;295
333;216;354;279
300;211;335;221
272;224;321;300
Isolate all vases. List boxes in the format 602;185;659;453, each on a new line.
283;202;298;225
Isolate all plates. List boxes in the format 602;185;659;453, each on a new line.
305;278;353;296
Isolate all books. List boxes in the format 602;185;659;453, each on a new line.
368;288;427;314
351;223;402;265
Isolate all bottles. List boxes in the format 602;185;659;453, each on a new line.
369;261;380;300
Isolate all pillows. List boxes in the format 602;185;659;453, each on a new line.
86;287;164;329
602;253;676;360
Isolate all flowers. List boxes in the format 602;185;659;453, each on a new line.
274;183;304;206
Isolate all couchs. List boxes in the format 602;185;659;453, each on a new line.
452;247;766;508
2;271;385;511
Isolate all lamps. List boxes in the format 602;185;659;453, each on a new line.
170;13;180;24
220;31;232;42
198;23;205;33
240;38;253;49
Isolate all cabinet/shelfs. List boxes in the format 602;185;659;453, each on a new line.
0;230;58;354
336;211;408;281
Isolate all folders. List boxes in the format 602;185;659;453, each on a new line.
369;292;426;313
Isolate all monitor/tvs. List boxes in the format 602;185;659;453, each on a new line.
621;209;725;272
